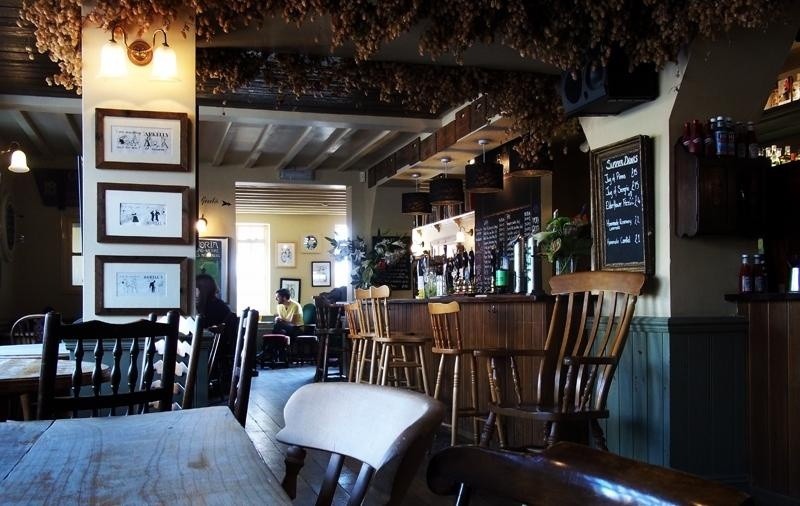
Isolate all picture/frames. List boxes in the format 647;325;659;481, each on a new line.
195;235;230;304
94;107;190;172
274;239;298;268
96;182;191;245
311;261;331;287
95;255;188;317
300;232;321;254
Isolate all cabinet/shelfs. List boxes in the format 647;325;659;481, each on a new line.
675;98;800;238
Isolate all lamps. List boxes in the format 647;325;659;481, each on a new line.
0;140;30;173
465;138;503;194
510;140;553;180
429;155;464;206
98;25;177;80
402;173;432;215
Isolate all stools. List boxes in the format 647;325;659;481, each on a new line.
262;333;291;369
423;299;507;450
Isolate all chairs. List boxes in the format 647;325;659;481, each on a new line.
429;442;603;504
469;274;647;452
0;305;259;429
263;273;432;397
271;379;447;504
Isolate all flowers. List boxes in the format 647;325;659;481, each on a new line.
324;227;410;288
532;206;589;272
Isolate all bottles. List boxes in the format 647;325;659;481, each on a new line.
759;144;800;167
753;253;768;293
682;115;759;158
739;255;753;292
789;267;799;292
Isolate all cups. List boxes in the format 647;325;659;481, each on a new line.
423;272;437;298
496;270;515;293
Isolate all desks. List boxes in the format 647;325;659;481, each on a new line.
257;320;275;332
1;404;293;505
724;292;800;498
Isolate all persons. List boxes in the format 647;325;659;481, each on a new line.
147;281;157;293
196;274;237;379
155;210;161;222
275;288;305;335
150;210;155;221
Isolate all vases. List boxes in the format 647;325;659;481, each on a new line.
555;253;577;275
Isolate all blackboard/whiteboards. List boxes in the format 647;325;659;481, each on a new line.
475;206;541;294
372;236;411;290
